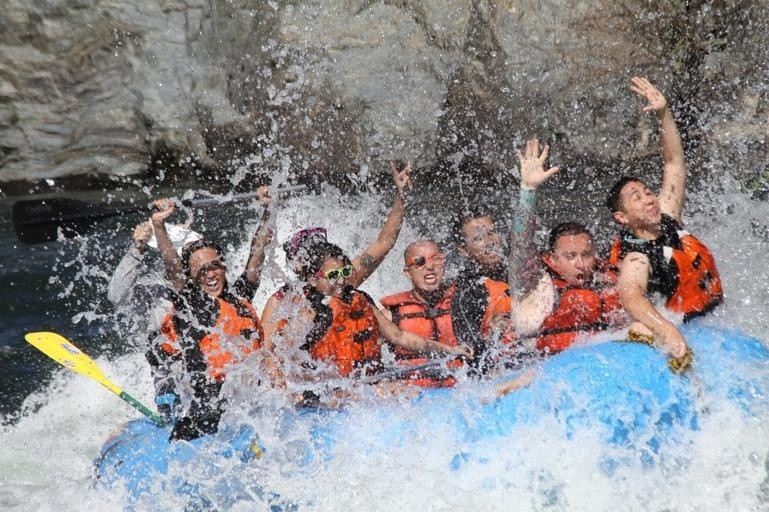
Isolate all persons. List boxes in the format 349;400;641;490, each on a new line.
602;74;731;379
449;208;537;380
148;180;276;453
105;215;237;425
253;158;420;412
274;239;474;419
506;133;627;361
381;238;470;392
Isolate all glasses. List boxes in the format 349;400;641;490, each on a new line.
291;227;325;254
182;238;215;257
406;254;444;267
313;266;353;282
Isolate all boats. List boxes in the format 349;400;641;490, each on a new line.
94;321;768;512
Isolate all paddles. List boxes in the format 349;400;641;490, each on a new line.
168;348;468;441
23;332;162;424
13;185;308;245
